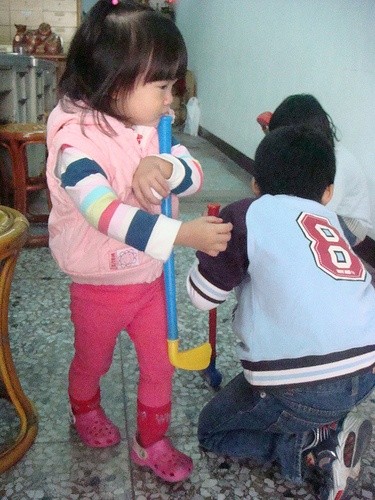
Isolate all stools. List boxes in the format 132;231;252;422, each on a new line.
1;207;38;474
0;124;52;246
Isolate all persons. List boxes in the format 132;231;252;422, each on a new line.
186;123;375;500
256;94;371;222
45;0;233;483
